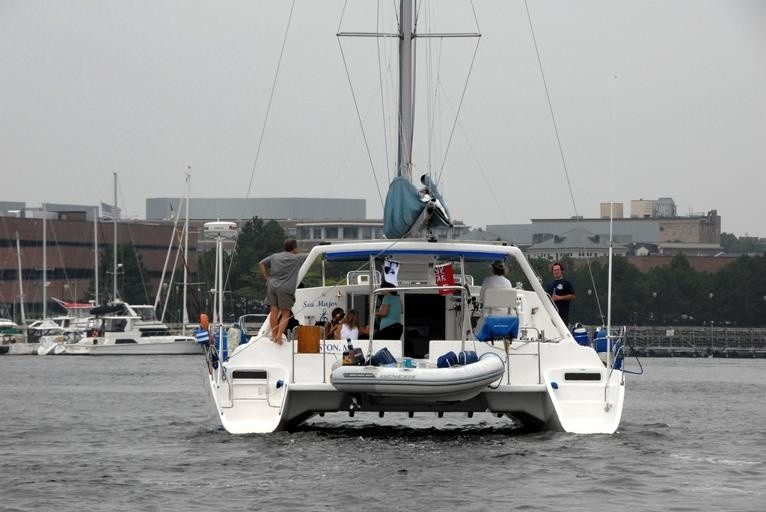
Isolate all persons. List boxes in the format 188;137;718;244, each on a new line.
323;307;345;340
546;261;576;328
404;329;420;357
373;281;404;340
336;309;369;340
472;259;513;336
257;237;308;346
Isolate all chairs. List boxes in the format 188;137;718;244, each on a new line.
475;289;519;346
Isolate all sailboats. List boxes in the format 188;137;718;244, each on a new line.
203;2;628;438
0;163;200;356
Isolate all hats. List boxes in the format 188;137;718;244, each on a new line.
490;259;506;270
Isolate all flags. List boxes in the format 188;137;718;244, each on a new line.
383;260;400;283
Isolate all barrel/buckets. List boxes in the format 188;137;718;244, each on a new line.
374;346;396;366
574;328;590;346
212;333;228;362
436;351;457;368
459;351;477;363
297;325;320;352
